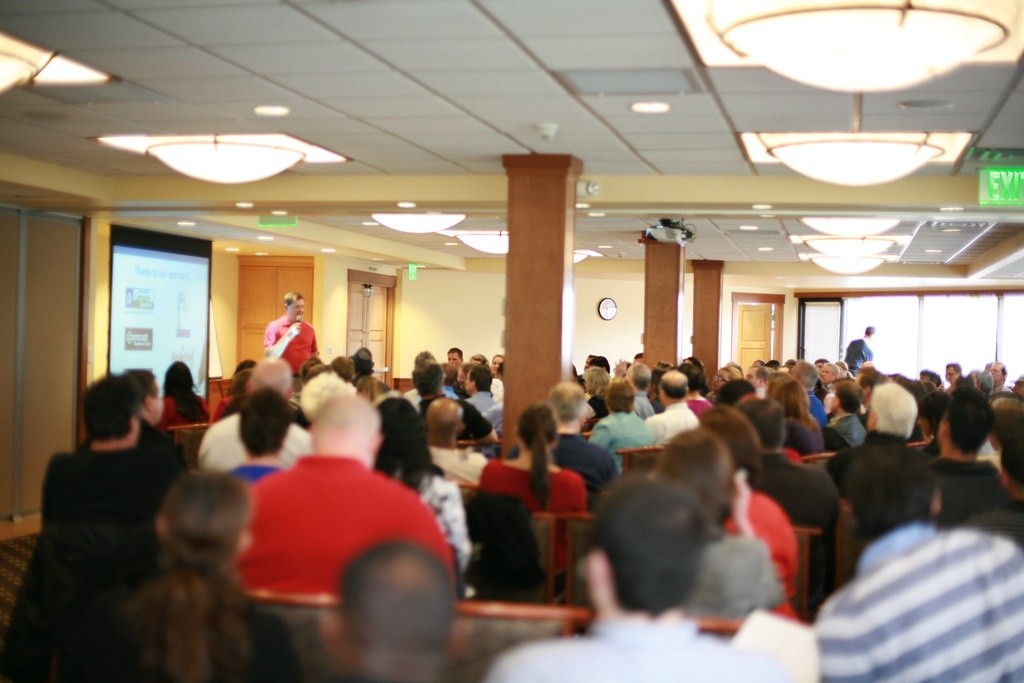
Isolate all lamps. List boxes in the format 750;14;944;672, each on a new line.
740;132;973;187
369;212;605;264
665;0;1024;92
789;215;913;276
0;30;110;96
98;132;350;184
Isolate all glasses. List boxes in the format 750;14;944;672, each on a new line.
713;374;728;382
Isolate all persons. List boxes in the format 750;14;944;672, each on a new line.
265;291;319;396
845;326;875;376
1;348;1024;682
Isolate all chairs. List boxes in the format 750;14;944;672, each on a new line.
6;424;930;683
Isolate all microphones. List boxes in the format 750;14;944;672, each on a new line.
297;317;302;324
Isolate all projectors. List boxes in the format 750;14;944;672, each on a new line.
645;226;687;245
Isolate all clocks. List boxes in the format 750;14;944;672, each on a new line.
599;298;617;320
